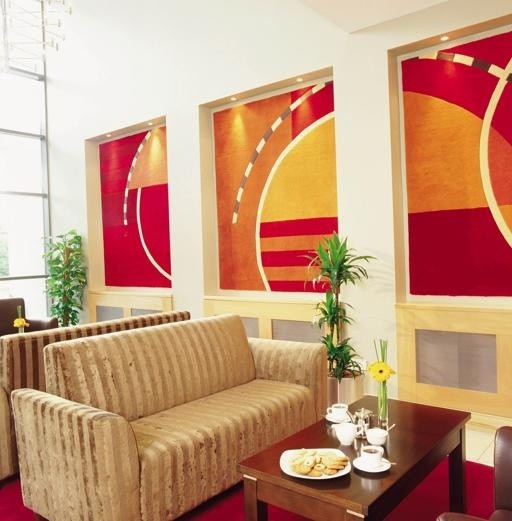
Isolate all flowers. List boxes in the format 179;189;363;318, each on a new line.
369;339;395;382
13;305;29;328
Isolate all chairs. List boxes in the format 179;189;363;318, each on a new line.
436;425;512;521
0;298;58;337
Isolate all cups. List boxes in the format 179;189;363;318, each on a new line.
365;428;388;446
326;404;349;420
359;446;385;468
332;423;364;447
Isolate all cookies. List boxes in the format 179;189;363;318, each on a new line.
290;449;348;477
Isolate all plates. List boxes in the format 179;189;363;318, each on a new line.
278;448;351;481
325;414;352;423
352;457;392;474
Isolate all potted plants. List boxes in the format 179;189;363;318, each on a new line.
301;232;377;406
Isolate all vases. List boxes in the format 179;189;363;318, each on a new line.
376;382;388;427
19;327;24;333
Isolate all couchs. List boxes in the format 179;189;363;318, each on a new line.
9;314;327;521
0;310;190;482
